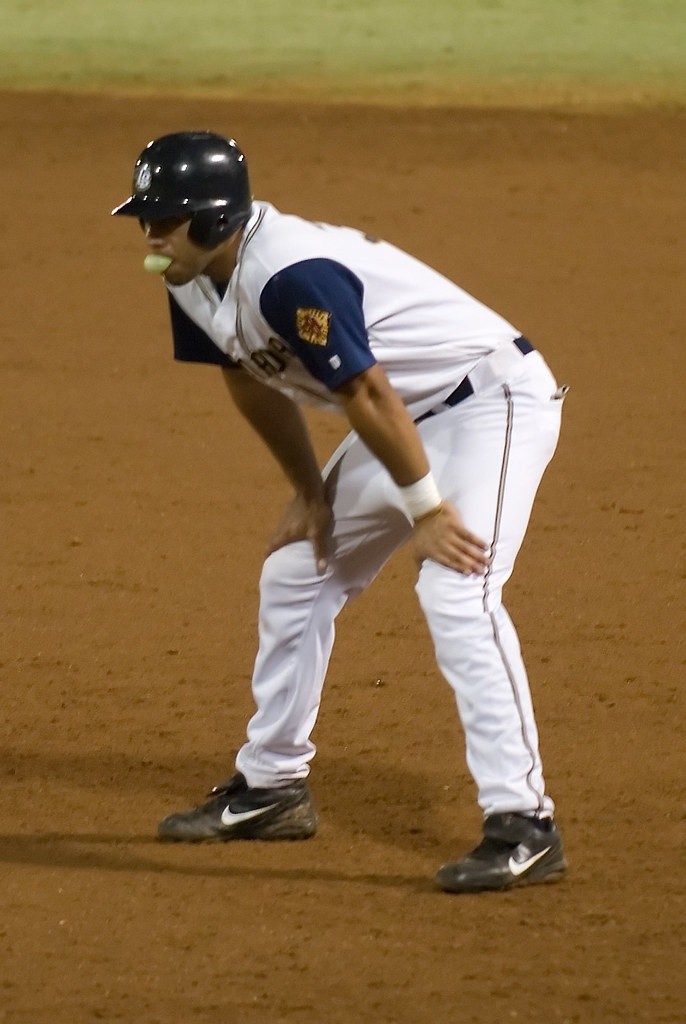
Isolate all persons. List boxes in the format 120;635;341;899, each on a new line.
112;130;566;894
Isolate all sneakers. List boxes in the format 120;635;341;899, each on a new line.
157;773;317;844
437;812;565;892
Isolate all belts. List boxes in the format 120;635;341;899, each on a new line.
413;336;533;422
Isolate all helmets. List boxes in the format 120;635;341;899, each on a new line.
111;130;252;251
